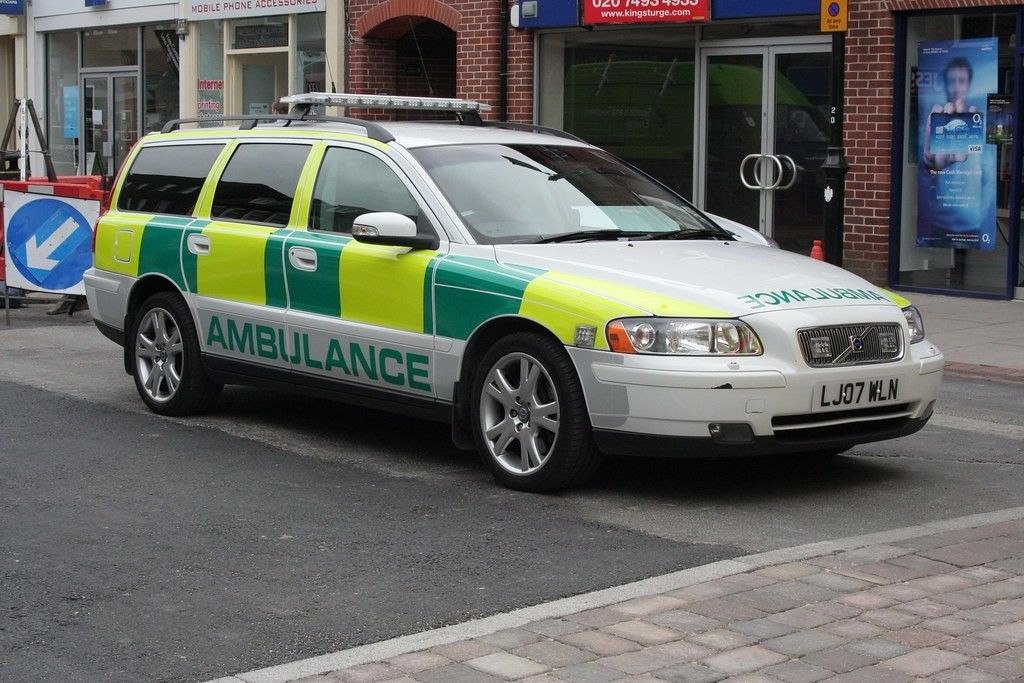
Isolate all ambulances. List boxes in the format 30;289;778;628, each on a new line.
83;91;945;496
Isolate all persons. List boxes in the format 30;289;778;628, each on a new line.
923;56;994;212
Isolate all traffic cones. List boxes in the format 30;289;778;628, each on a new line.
809;239;825;262
